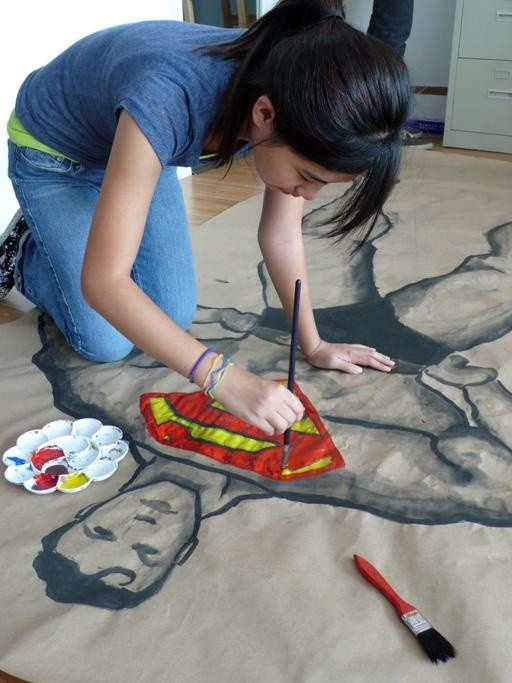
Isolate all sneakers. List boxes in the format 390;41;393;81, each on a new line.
1;207;31;302
400;128;435;151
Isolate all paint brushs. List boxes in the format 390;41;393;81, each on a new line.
281;280;302;471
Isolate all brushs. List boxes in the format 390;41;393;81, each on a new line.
354;554;455;665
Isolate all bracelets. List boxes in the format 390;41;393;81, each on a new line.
189;347;235;401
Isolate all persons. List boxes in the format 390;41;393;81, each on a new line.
302;0;434;149
32;174;511;610
0;0;418;438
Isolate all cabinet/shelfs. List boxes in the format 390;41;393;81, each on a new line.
442;0;512;154
